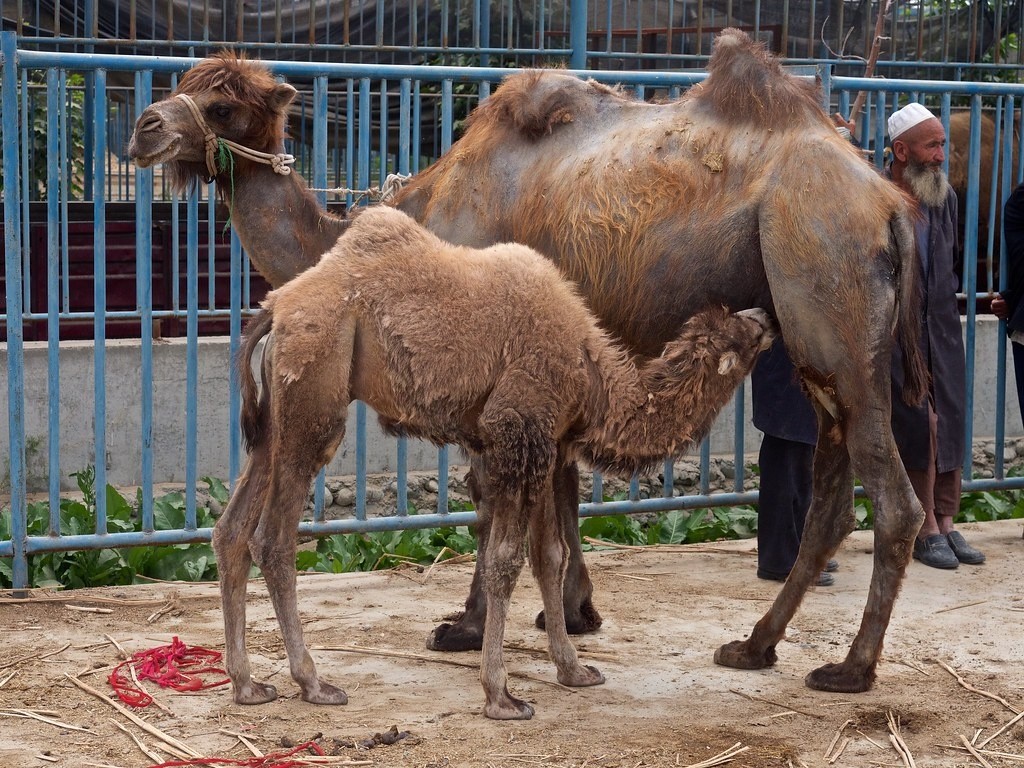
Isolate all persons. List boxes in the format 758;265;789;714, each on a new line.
990;181;1024;428
877;101;987;572
750;111;862;589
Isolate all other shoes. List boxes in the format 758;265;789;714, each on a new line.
822;559;839;572
757;567;834;586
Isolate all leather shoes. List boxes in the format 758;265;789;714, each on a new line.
945;531;986;563
911;534;959;569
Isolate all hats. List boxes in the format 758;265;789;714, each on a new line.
887;103;935;141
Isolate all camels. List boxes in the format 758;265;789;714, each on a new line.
935;105;1024;230
214;204;778;724
126;25;926;694
